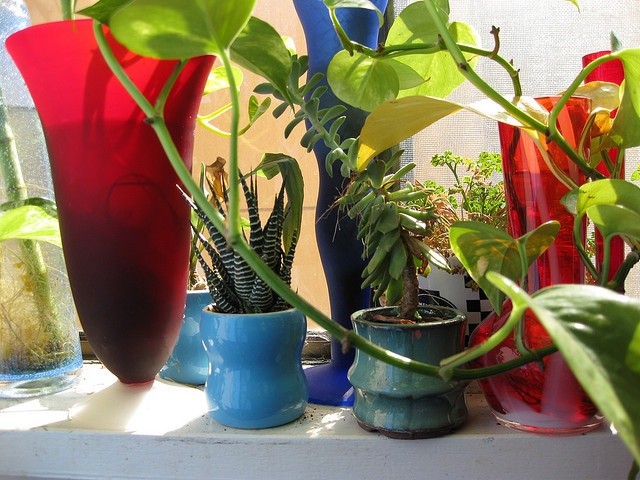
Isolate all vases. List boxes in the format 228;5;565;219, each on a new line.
293;0;393;406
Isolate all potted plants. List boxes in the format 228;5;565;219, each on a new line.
2;0;215;437
162;163;217;385
409;149;515;339
333;0;629;435
175;163;311;431
0;0;86;400
254;50;469;442
